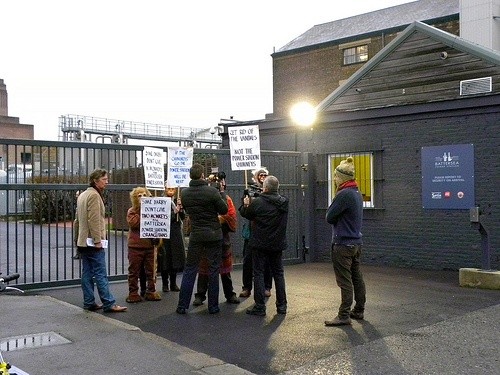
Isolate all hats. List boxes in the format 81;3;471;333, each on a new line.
336;157;354;177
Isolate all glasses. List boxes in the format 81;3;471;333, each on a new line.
259;174;267;176
138;194;146;197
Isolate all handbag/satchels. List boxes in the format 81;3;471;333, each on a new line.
242;221;250;239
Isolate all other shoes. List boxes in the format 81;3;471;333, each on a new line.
247;303;266;316
209;308;219;314
162;284;169;292
239;287;251;296
176;307;186;314
105;303;127;312
277;308;286;314
93;303;104;309
192;296;204;305
264;288;271;296
349;310;365;319
170;283;179;291
324;316;352;326
227;293;241;304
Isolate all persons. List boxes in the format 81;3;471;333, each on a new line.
192;171;240;306
247;174;287;316
326;156;366;326
139;181;187;298
71;190;84;259
176;163;228;314
77;169;127;313
240;168;272;297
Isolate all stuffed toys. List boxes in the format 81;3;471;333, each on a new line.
125;187;162;303
